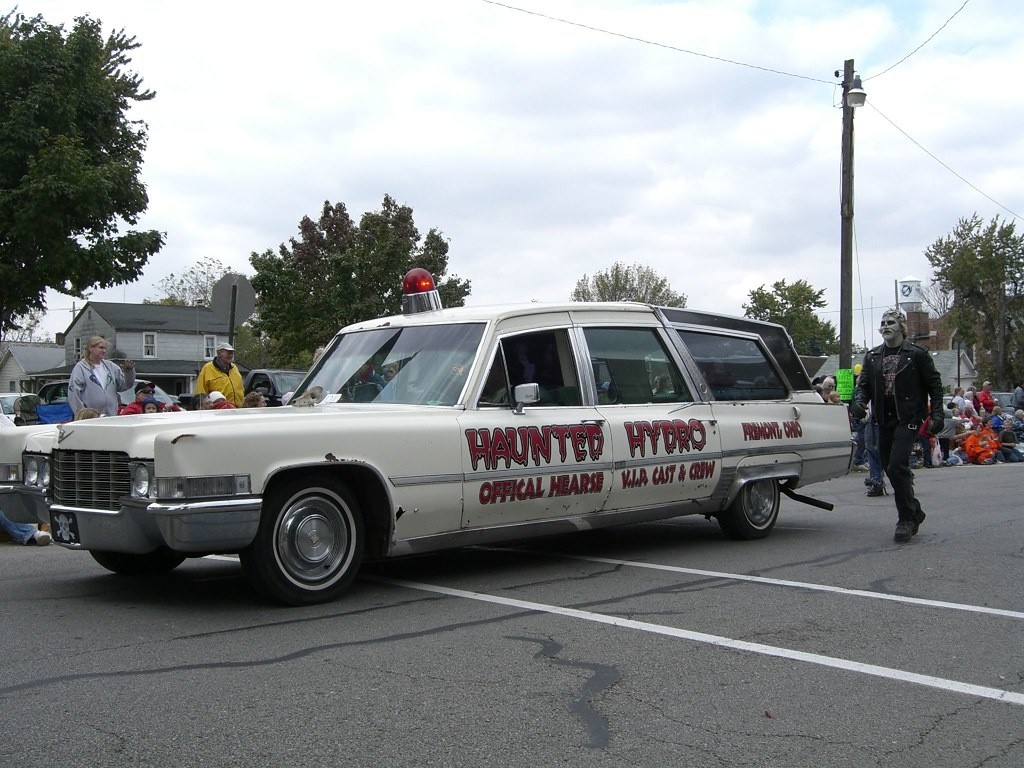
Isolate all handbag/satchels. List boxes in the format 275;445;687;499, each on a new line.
930;437;942;467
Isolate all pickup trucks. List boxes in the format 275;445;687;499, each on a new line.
179;368;308;411
9;267;857;606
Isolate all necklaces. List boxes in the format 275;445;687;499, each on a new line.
882;346;903;377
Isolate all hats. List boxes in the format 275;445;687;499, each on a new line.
216;342;235;351
143;397;157;405
135;382;155;394
208;391;226;403
882;308;906;324
982;381;992;387
1015;409;1024;416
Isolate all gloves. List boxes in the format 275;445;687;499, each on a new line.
930;414;944;435
853;393;869;420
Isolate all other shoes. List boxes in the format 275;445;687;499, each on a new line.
894;518;918;541
854;465;868;471
911;508;926;535
925;464;933;468
864;462;870;467
36;530;51;547
867;483;883;496
864;477;877;485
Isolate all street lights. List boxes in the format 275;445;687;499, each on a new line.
840;75;867;406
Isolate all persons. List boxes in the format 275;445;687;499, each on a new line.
314;347;349;403
387;362;399;379
74;408;100;421
599;382;610;403
814;374;870;475
0;510;53;546
488;339;526;403
919;381;1024;468
192;391;267;410
68;336;136;416
121;382;181;415
358;361;385;388
652;376;667;395
193;342;245;408
854;309;946;544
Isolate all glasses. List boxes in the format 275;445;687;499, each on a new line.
386;370;393;374
140;390;155;394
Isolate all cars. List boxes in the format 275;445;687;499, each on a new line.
38;379;188;414
